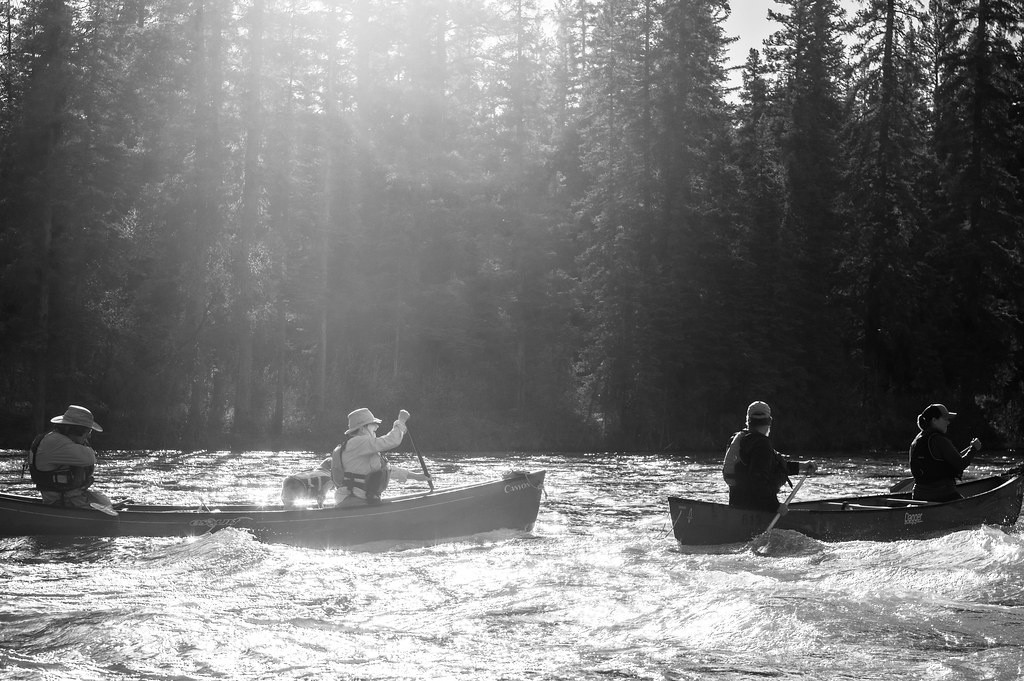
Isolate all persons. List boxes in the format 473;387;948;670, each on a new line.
330;408;432;508
908;403;982;504
722;401;818;514
28;403;113;511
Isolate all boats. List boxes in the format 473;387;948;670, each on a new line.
1;465;548;555
665;461;1023;544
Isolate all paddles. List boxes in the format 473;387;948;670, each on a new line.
90;498;135;516
760;468;808;533
405;420;435;490
890;446;970;493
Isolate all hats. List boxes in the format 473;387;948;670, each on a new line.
50;405;104;432
923;404;957;420
344;407;382;434
747;400;771;418
